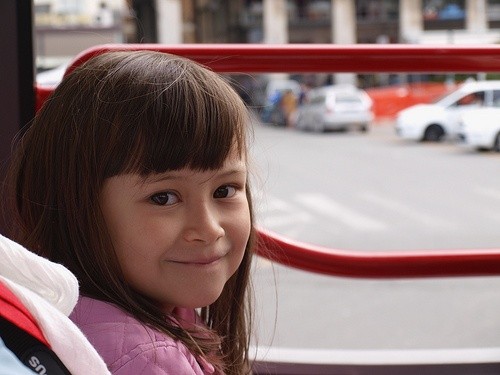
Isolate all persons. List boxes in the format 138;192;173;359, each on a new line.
10;51;256;375
262;80;312;129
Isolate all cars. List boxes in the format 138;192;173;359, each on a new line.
260;77;299;125
459;105;500;153
395;78;499;141
293;83;375;133
